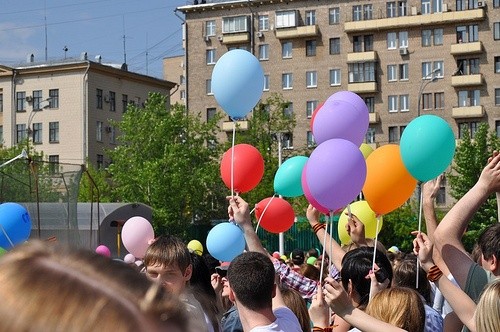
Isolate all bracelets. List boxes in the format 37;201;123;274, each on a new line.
427;265;443;282
312;222;326;234
313;325;338;332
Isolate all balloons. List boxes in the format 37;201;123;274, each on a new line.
206;223;245;262
96;245;111;256
301;90;369;215
362;144;418;217
212;48;264;119
221;144;265;193
0;202;33;252
122;216;154;258
400;114;456;184
254;197;295;234
188;240;203;256
273;155;309;197
338;201;383;245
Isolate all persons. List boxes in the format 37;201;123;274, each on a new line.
0;151;500;332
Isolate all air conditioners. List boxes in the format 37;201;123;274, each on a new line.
26;96;33;102
256;32;262;38
104;95;110;103
217;36;223;41
106;127;112;132
399;47;408;56
204;36;209;41
477;1;486;8
